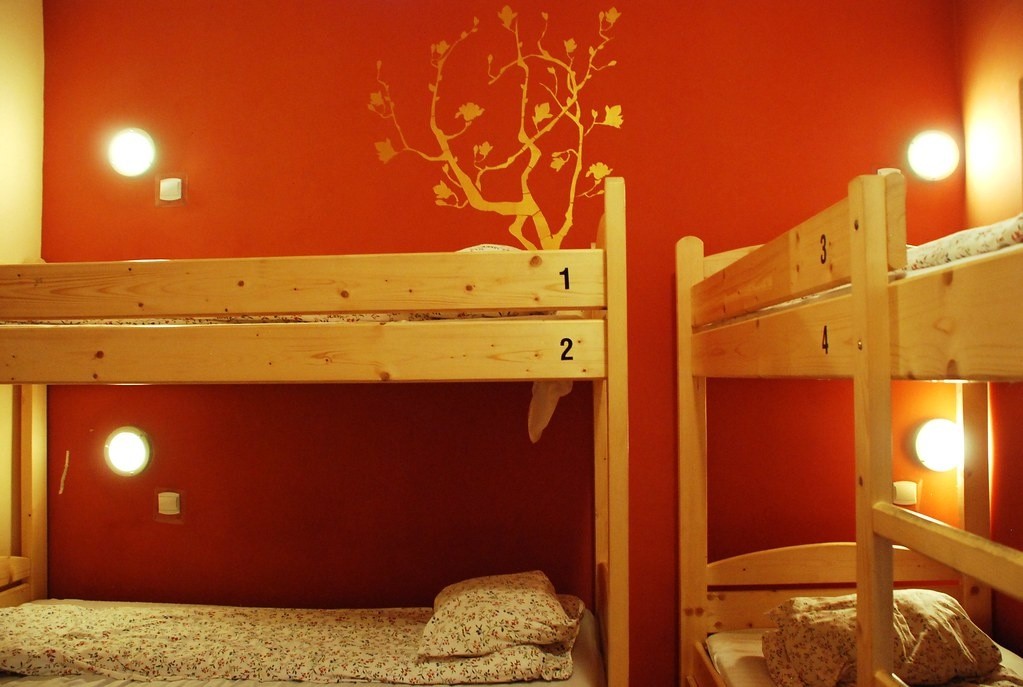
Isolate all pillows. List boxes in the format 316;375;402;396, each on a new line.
761;630;807;687
417;569;579;657
778;587;1002;687
451;243;527;254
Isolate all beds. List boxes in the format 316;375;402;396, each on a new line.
672;168;1023;687
0;179;631;687
